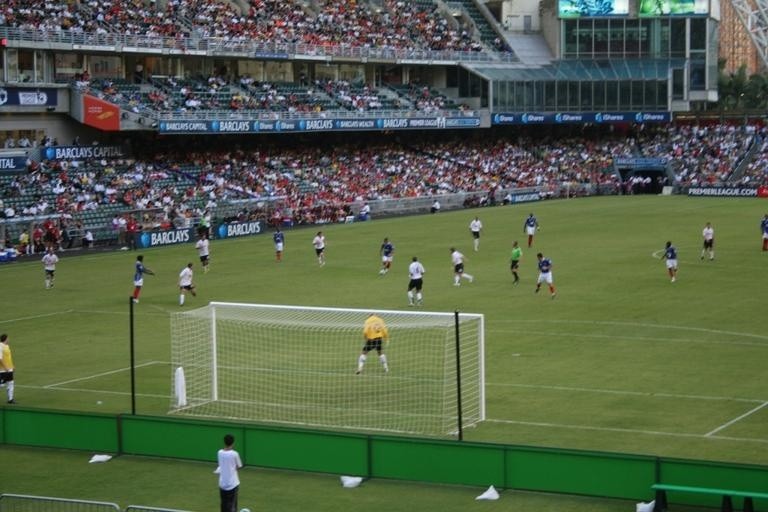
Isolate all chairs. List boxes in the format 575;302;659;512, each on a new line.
0;1;767;249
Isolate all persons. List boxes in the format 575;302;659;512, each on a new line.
535;252;555;297
1;3;768;260
132;255;154;304
355;313;390;374
510;241;523;285
194;234;212;272
406;256;425;307
0;333;17;404
313;232;326;266
450;247;473;286
42;246;58;289
216;434;243;512
177;262;196;307
379;238;395;274
661;241;677;282
